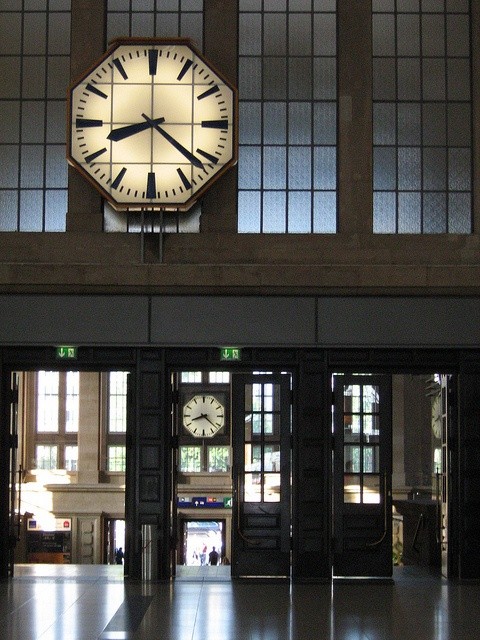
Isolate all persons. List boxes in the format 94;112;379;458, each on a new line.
222;554;227;563
199;544;207;565
209;547;218;565
117;548;123;564
193;552;198;559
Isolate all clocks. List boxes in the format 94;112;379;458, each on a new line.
178;384;233;446
68;38;238;211
